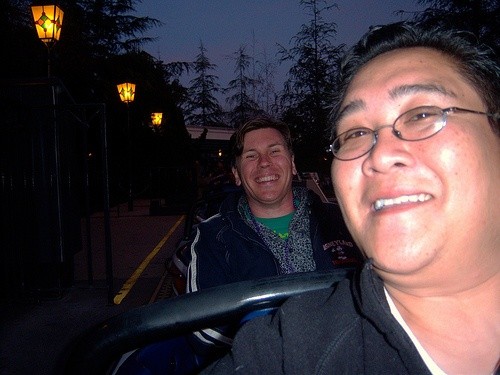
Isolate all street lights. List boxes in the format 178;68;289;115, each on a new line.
151;107;164;213
29;0;66;265
116;81;136;209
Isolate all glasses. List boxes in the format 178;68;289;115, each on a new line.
325;106;498;162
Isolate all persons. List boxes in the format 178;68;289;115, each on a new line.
111;114;366;375
200;20;500;375
